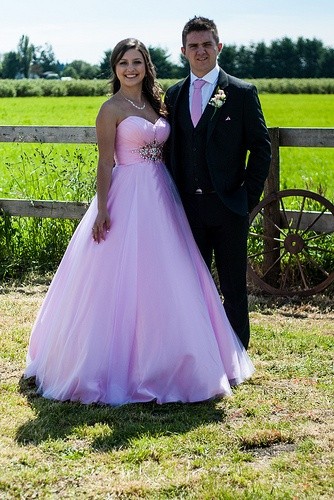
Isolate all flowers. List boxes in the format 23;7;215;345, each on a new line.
208;85;226;120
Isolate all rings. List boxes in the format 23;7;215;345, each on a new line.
93;228;95;230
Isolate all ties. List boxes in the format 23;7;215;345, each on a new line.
191;79;206;127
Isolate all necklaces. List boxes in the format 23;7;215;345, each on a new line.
125;98;146;110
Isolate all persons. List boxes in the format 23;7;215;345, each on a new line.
162;18;272;350
20;40;256;405
